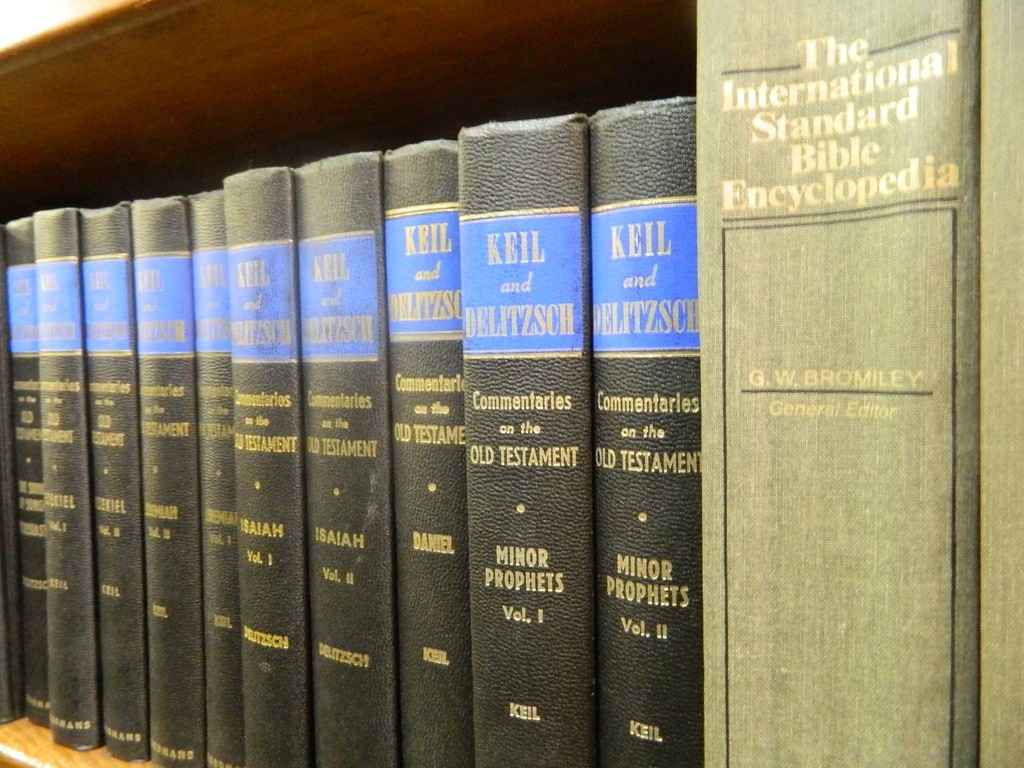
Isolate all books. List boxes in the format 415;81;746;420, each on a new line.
0;0;980;767
